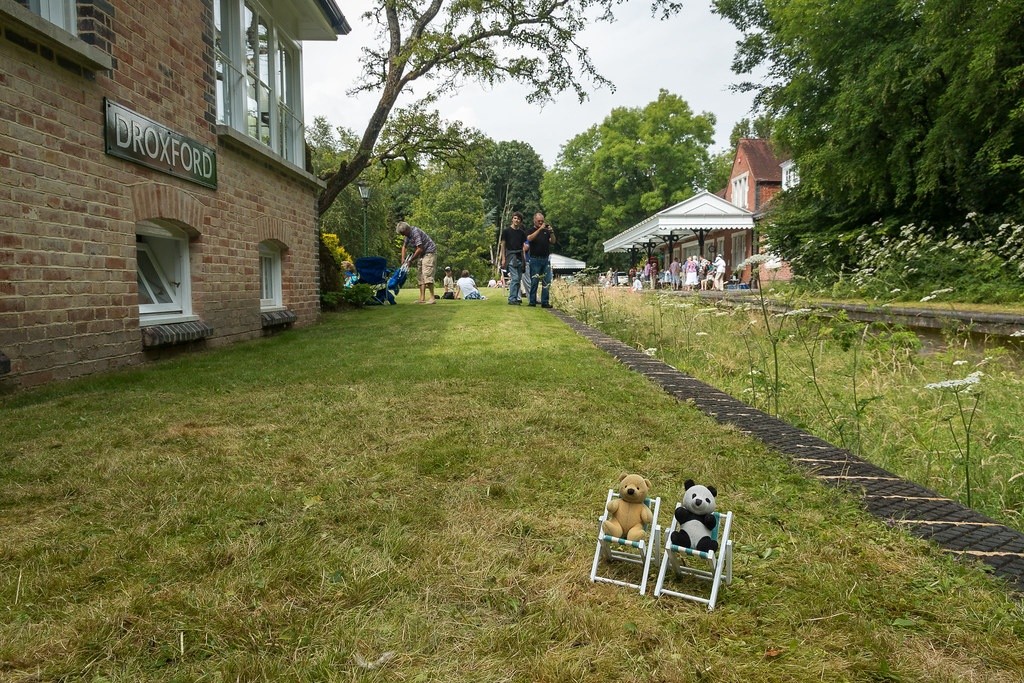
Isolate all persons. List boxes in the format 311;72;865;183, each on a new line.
455;270;481;300
501;211;527;305
516;240;541;304
488;277;503;288
443;266;455;293
606;267;618;287
396;221;437;304
527;212;557;308
628;254;726;292
341;260;359;285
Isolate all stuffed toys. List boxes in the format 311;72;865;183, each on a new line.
602;472;653;541
671;479;719;552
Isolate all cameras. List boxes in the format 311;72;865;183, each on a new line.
545;223;549;228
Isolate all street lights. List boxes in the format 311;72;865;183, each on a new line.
358;176;373;258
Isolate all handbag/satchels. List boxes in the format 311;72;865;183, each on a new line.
440;291;454;299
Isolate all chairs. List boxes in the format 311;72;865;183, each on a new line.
346;257;392;306
654;502;733;611
590;488;661;596
500;269;511;297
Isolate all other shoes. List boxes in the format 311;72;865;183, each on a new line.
527;302;537;307
415;300;425;303
509;301;521;305
541;303;553;308
426;300;436;304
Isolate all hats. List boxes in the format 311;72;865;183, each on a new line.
445;267;451;272
715;257;720;262
